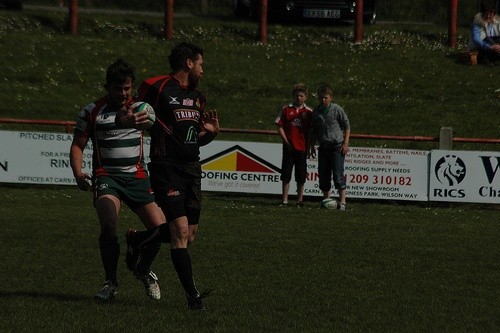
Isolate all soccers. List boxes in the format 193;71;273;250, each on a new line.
129;101;155;130
322;197;337;210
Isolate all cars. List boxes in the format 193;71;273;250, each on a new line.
233;0;377;25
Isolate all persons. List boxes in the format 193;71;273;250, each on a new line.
311;85;350;208
275;83;313;207
466;5;500;65
115;43;219;310
70;59;167;300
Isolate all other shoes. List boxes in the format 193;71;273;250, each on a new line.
338;203;346;211
279;202;289;207
296;202;303;208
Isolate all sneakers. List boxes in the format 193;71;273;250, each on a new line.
186;289;211;311
142;271;161;301
126;227;139;270
95;281;119;300
